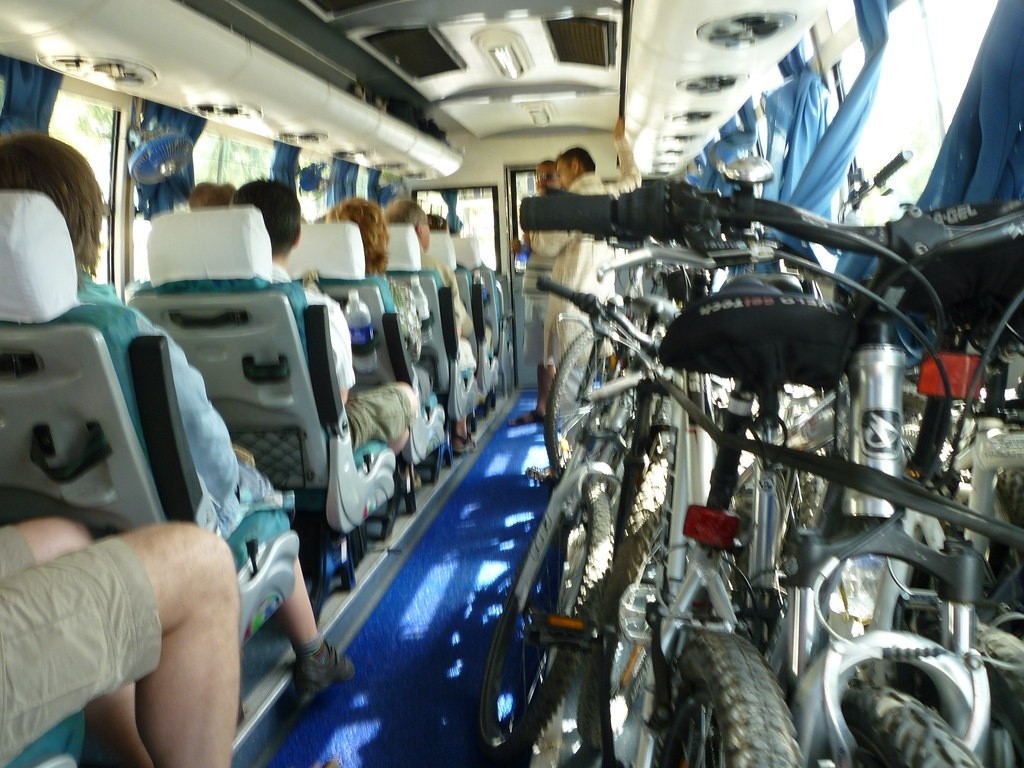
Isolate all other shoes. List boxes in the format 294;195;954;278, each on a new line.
528;466;557;484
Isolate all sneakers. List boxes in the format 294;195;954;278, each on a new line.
295;640;356;694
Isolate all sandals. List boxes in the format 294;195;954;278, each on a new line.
510;408;546;426
452;433;476;453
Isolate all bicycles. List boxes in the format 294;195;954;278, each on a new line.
477;157;1024;768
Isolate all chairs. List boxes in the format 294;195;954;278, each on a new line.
0;189;508;768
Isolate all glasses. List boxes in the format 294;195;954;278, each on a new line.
536;172;558;181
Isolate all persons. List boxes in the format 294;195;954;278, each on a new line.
511;117;641;426
0;135;493;768
